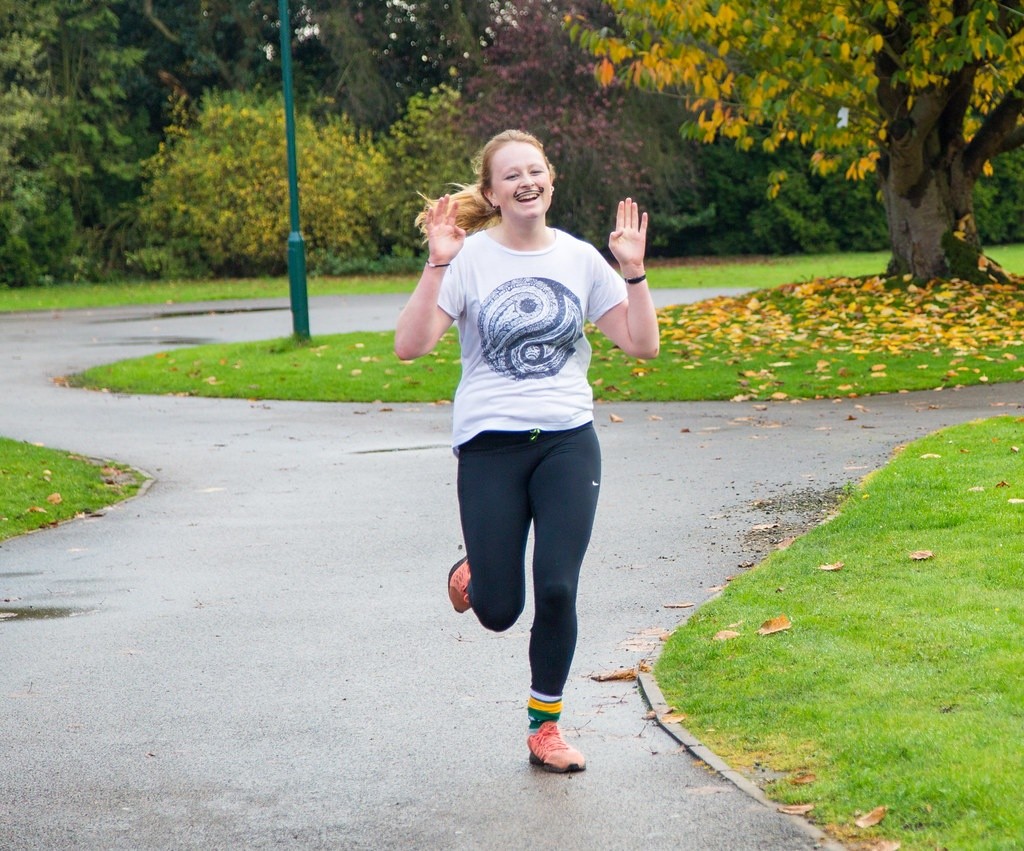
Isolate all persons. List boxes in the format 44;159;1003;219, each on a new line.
394;128;661;774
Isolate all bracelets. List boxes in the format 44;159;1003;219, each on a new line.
625;274;646;284
427;260;450;268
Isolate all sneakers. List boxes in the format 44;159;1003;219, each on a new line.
526;722;586;775
448;555;472;613
